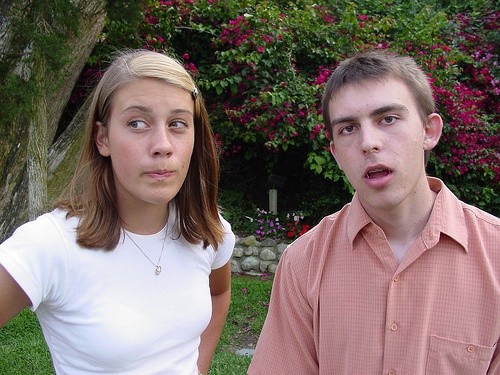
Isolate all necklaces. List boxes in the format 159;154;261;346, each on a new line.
119;220;168;275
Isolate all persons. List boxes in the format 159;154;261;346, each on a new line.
0;51;235;375
246;53;500;375
